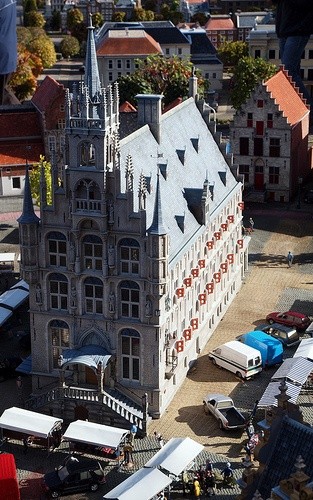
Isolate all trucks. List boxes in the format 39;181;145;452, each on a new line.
202;393;247;432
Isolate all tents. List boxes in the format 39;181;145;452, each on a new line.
143;437;204;477
61;420;129;466
257;337;313;408
0;406;64;439
103;466;172;500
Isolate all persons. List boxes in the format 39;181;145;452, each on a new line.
244;432;259;463
286;251;294;269
191;460;214;497
221;463;232;484
249;218;254;232
130;422;138;443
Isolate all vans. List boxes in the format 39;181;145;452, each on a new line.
207;340;263;381
236;330;284;370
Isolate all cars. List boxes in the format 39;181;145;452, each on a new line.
43;456;107;499
265;311;310;333
253;323;300;349
